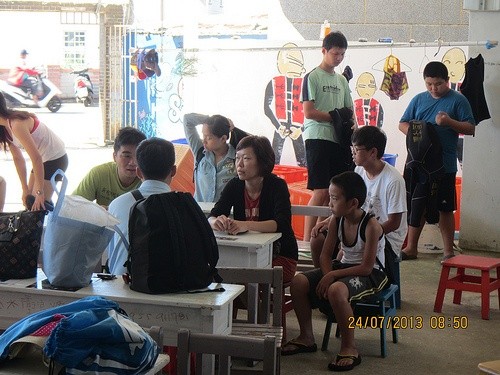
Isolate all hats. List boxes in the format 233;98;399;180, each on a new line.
130;49;161;80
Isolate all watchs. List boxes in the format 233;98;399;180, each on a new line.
36;191;43;195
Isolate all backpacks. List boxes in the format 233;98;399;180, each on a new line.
125;190;220;294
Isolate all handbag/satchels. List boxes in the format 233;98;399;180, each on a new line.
44;169;129;288
0;209;48;282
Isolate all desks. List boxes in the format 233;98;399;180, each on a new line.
214;228;284;367
0;273;246;375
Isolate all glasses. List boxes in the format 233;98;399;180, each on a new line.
350;146;368;152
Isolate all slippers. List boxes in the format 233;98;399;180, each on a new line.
328;353;361;371
401;250;417;261
279;338;317;356
443;255;455;261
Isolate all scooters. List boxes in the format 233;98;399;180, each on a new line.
70;62;94;107
0;72;63;113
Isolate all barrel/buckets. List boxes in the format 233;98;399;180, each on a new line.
454;176;461;231
382;153;399;167
271;163;309;184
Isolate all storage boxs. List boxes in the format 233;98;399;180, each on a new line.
168;137;315;236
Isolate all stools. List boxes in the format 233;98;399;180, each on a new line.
320;285;401;358
432;255;500;314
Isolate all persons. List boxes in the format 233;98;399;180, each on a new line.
303;31;357;243
0;91;69;212
207;136;298;298
71;127;147;210
185;113;252;203
394;61;475;264
107;137;177;276
278;172;390;371
310;126;408;269
8;50;41;100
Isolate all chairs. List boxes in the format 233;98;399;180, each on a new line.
135;206;333;368
